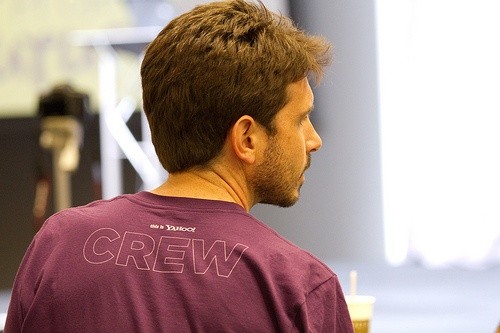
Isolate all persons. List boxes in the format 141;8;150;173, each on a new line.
2;2;355;333
